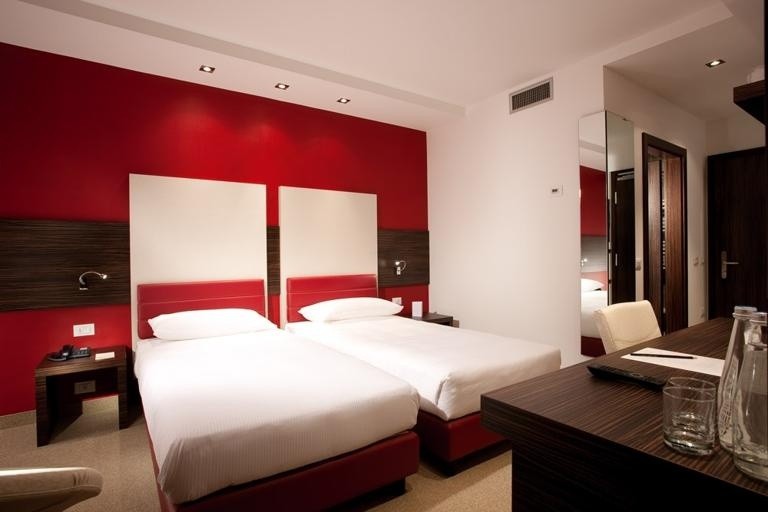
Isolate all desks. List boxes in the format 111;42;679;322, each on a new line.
481;316;767;509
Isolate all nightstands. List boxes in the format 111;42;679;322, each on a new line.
35;346;129;447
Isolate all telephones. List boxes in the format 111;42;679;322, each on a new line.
47;344;90;361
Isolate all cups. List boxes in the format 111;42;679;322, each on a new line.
666;376;717;443
661;387;717;457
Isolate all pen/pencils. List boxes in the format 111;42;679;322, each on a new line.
630;353;696;359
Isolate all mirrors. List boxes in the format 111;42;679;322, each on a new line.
579;112;634;357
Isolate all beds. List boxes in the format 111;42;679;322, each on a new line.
287;275;561;477
135;280;420;511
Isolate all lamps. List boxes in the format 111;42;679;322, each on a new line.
394;259;403;276
78;270;106;291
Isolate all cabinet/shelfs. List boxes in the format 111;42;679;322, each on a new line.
406;313;452;326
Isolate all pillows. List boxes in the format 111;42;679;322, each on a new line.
147;308;277;341
298;296;403;324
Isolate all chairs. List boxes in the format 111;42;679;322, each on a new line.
596;299;660;353
